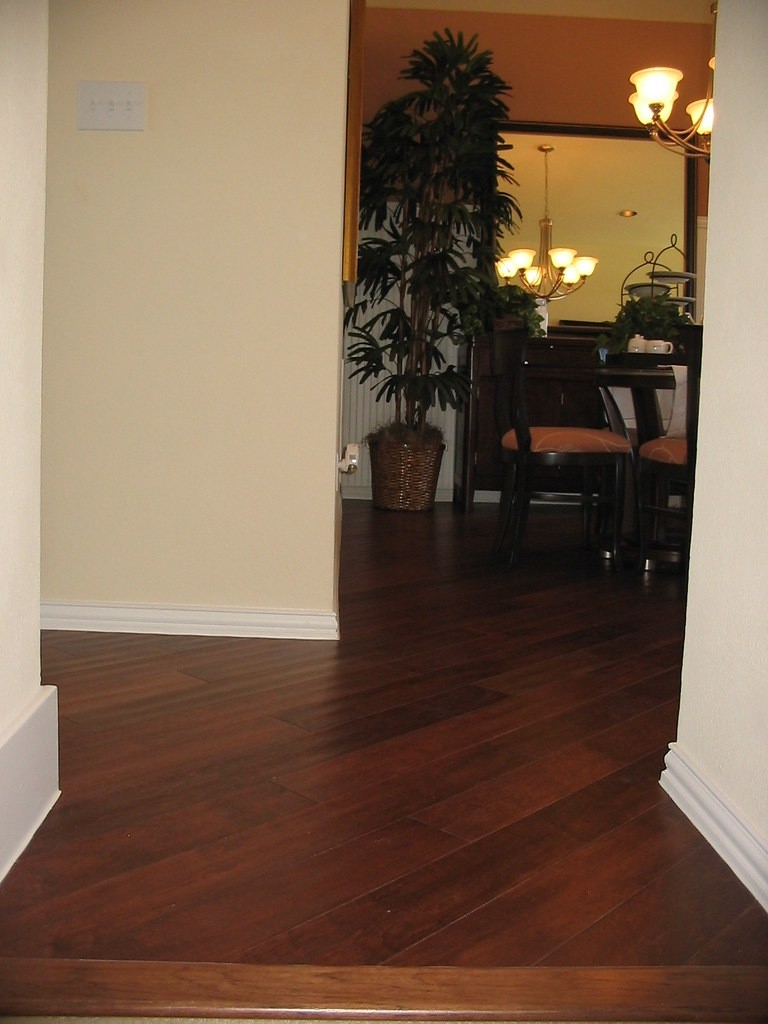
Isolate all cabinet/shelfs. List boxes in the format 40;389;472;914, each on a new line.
452;314;703;514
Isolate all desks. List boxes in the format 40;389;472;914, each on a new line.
591;352;704;571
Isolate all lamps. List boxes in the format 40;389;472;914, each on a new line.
495;144;599;302
628;0;718;164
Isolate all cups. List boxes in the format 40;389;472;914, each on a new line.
646;340;674;354
628;335;647;353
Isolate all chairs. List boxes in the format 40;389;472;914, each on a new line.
491;316;630;566
637;347;704;572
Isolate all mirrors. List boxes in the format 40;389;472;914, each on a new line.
481;119;700;331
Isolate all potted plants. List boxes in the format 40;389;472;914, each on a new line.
344;26;522;512
458;285;546;339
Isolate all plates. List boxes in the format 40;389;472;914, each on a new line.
646;271;697;284
661;297;697;307
624;283;672;298
